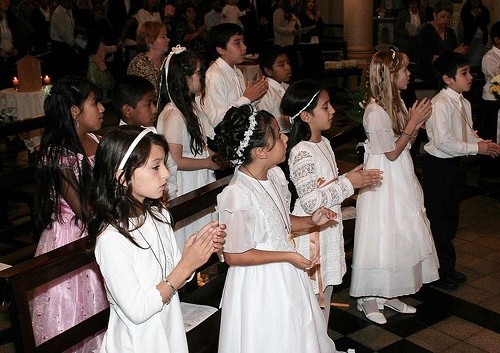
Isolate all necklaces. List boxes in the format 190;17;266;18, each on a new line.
124;207;168;280
444;88;483;142
308;134;340;186
242;163;298;251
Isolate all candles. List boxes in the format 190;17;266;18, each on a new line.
43;76;51;83
14;78;18;90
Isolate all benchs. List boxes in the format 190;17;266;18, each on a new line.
283;40;364;95
1;122;367;353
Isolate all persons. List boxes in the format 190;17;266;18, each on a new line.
26;76;121;353
155;46;214;200
283;79;353;352
1;0;500;138
88;124;227;353
420;52;500;291
213;104;350;353
204;22;250;139
347;49;442;326
255;45;299;164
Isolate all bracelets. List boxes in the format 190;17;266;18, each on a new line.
160;276;181;297
397;125;410;146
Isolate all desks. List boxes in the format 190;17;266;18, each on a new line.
237;61;264;87
0;85;51;121
